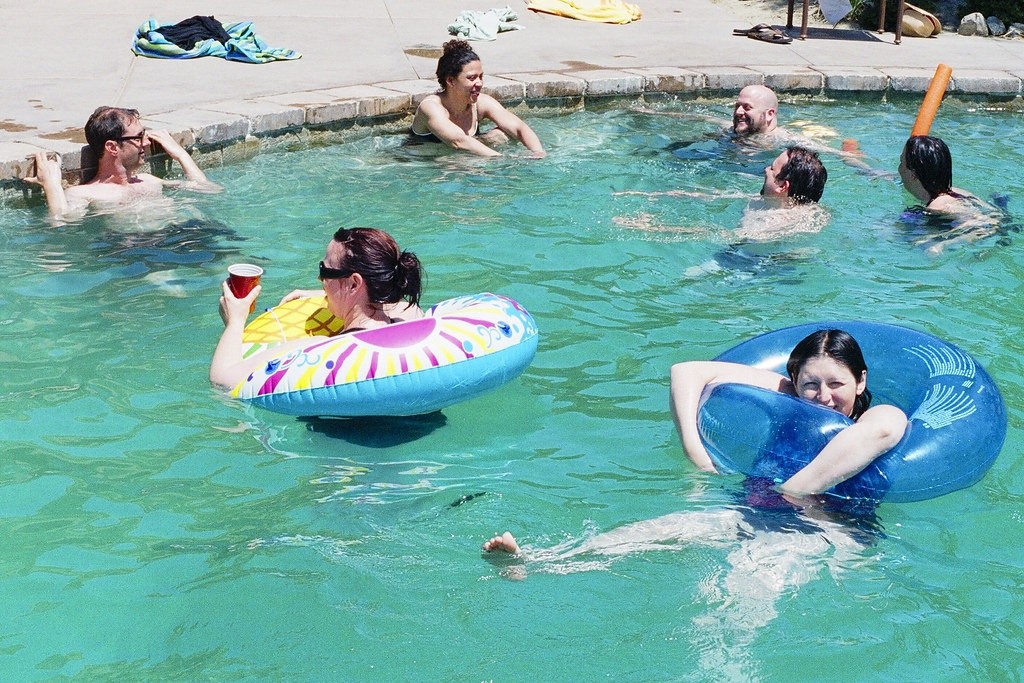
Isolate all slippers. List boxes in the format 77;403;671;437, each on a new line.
733;22;793;43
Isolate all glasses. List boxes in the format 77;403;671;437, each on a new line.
115;129;146;141
319;261;352;282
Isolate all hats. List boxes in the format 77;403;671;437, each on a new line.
901;2;941;38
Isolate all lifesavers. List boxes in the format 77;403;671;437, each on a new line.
220;292;538;419
702;317;1010;506
842;63;951;155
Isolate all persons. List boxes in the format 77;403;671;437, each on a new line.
758;146;826;204
411;41;543;158
22;106;205;212
210;227;424;387
898;135;976;212
483;329;908;555
734;85;782;136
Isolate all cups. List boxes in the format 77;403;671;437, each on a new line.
227;263;264;313
